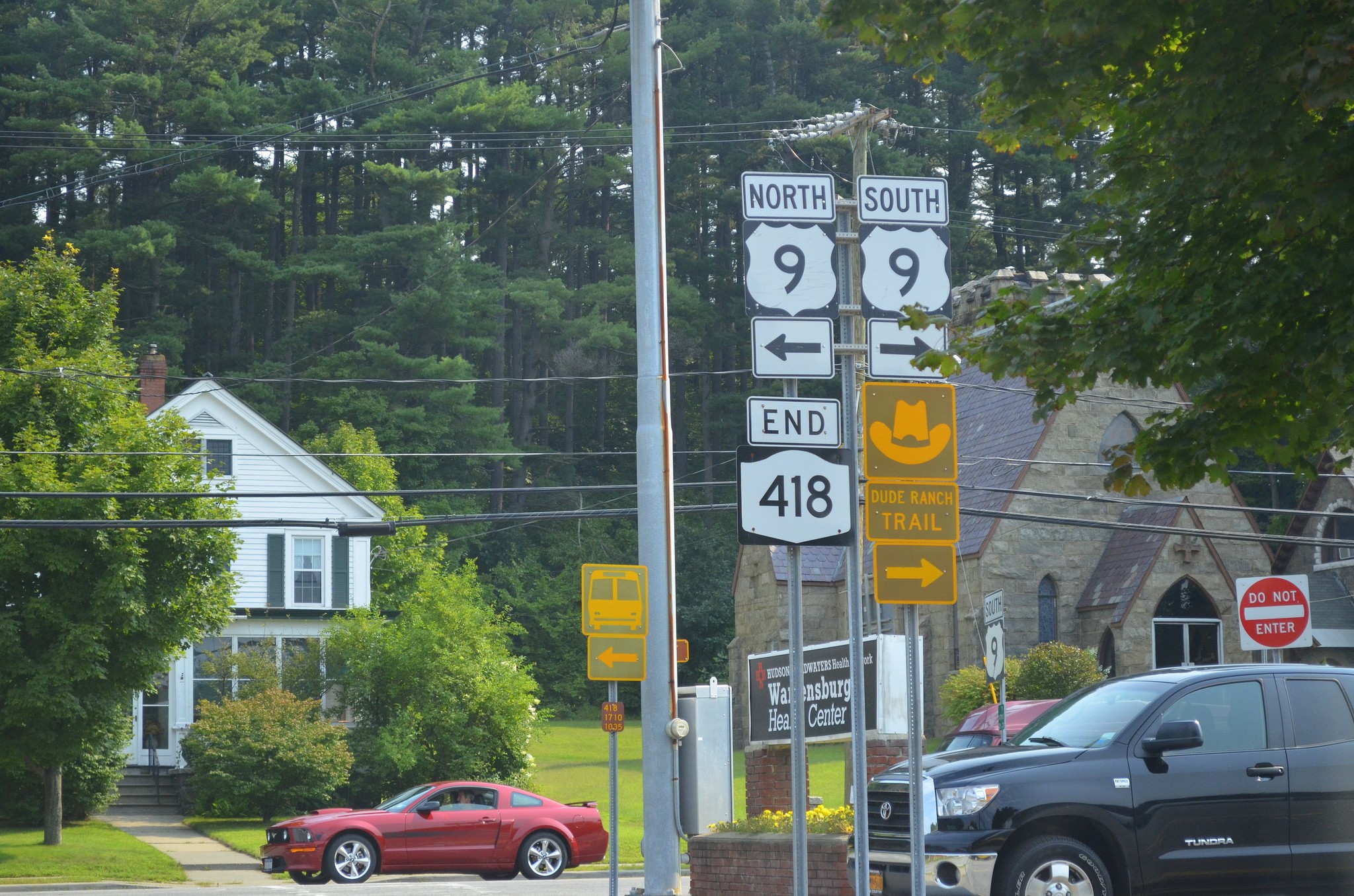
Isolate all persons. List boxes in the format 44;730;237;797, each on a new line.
143;717;158;737
457;789;475;810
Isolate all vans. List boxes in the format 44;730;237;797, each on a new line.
934;698;1163;753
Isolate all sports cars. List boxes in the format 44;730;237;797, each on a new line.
258;781;609;885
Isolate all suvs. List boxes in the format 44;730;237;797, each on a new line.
846;663;1354;896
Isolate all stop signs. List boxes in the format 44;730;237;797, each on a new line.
1236;574;1313;651
